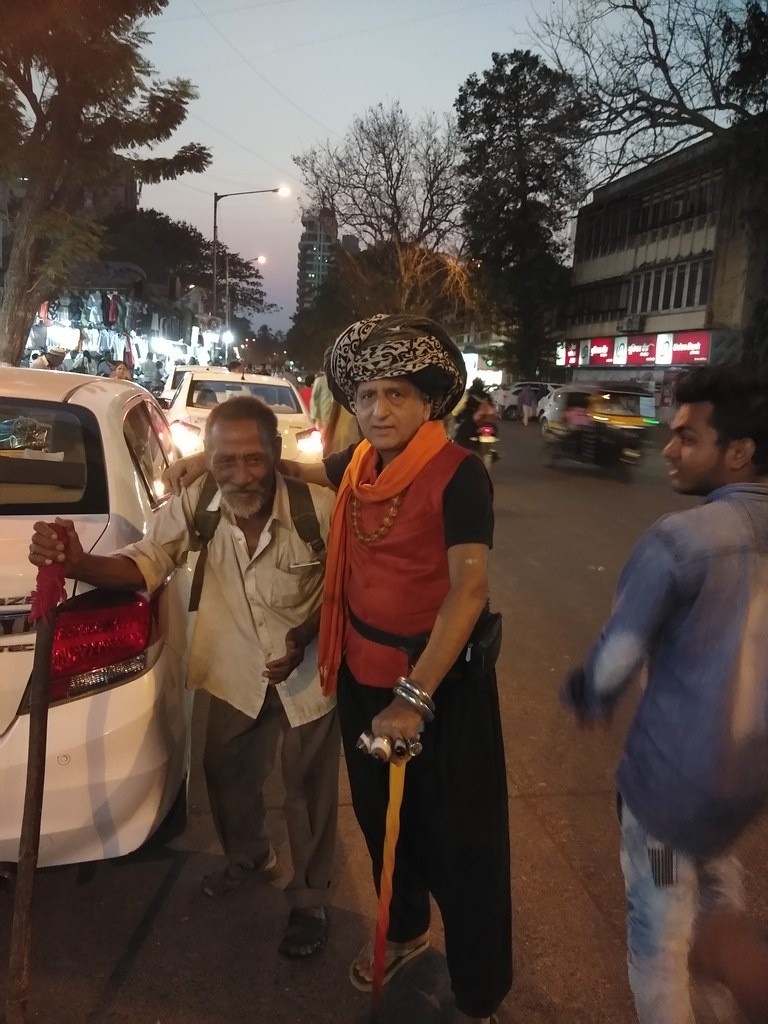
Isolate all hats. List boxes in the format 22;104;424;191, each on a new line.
325;314;468;421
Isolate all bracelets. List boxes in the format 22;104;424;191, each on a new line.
397;676;435;712
393;686;433;721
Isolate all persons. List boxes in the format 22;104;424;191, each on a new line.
33;349;162;390
455;377;491;442
175;356;297;387
300;376;313;407
160;313;512;1024
27;396;341;956
537;385;549;401
559;363;768;1024
495;384;507;417
518;384;536;426
311;376;358;450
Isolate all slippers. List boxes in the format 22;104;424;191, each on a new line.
203;851;277;897
276;902;328;959
348;926;432;994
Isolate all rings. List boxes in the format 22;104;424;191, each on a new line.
370;735;392;761
356;730;373;753
395;739;406;753
407;737;422;756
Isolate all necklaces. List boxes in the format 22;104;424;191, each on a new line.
349;489;406;545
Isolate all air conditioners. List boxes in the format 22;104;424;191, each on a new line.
622;316;639;331
672;200;683;218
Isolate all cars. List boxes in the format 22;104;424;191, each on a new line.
152;364;324;465
482;380;663;469
0;358;207;873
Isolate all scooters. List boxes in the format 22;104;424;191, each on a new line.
453;414;500;471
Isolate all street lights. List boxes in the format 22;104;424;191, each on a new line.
225;255;266;365
211;185;292;364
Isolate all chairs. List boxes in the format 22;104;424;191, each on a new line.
196;389;217;405
253;387;272;406
